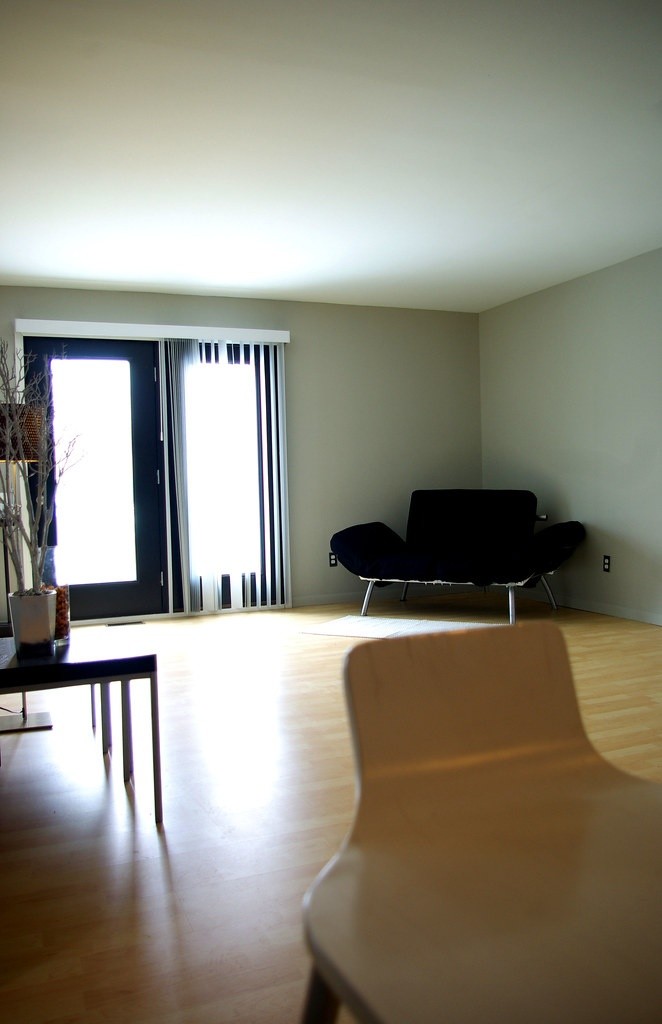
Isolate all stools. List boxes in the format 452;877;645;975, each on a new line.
300;623;662;1024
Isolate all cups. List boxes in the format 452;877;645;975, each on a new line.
37;546;70;645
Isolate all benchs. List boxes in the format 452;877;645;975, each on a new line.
329;488;587;624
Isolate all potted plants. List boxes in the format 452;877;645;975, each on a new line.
0;333;88;662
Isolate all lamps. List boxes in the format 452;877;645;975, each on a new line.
0;403;47;552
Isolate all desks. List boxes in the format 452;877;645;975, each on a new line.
1;629;165;824
